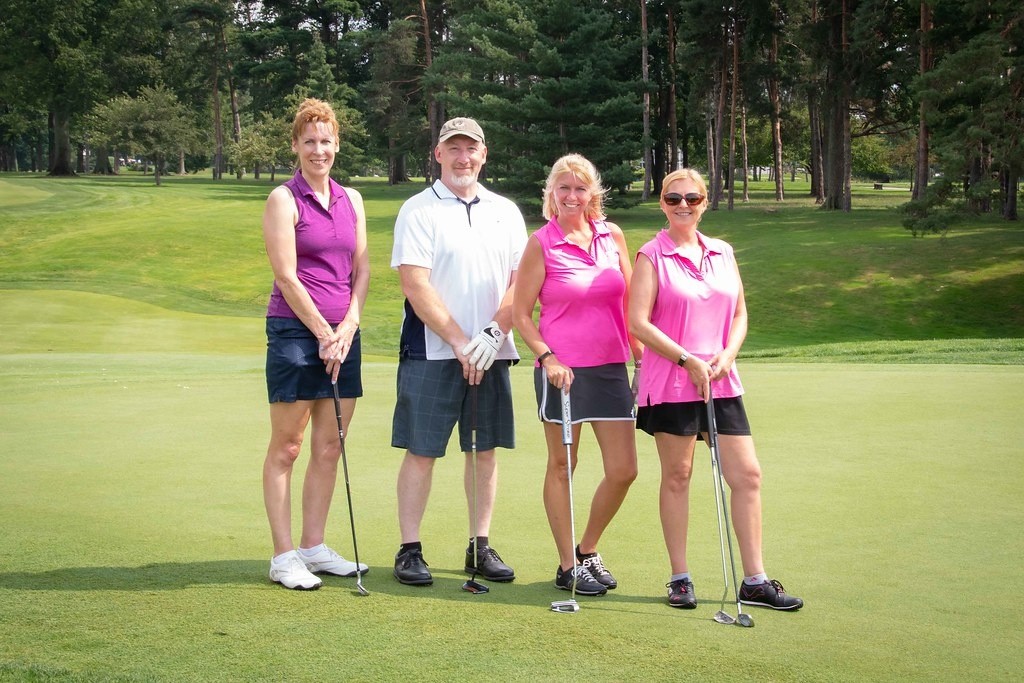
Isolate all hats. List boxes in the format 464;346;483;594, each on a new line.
439;117;485;145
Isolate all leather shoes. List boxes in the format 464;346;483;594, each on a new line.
465;547;515;581
393;547;433;585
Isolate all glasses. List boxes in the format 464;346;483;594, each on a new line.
663;193;705;207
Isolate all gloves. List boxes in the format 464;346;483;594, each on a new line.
463;321;508;371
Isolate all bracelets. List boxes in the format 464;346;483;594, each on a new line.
635;360;642;365
678;351;690;367
538;350;555;366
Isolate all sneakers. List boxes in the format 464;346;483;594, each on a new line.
555;560;607;595
576;546;618;589
739;580;803;610
268;555;322;590
666;577;697;608
296;544;369;577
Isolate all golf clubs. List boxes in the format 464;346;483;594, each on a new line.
461;383;490;594
704;374;755;629
330;371;370;597
547;383;580;614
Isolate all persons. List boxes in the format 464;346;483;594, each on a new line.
628;167;803;613
512;155;643;596
263;96;370;591
391;117;529;586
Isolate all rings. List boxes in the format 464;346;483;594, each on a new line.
709;360;715;365
